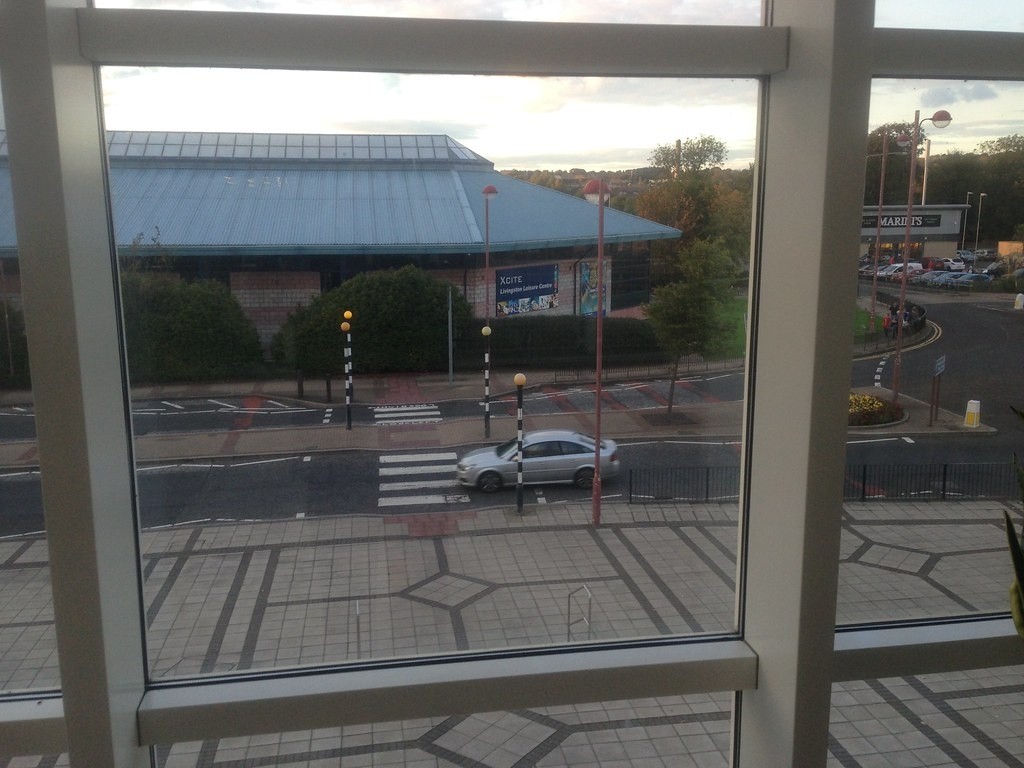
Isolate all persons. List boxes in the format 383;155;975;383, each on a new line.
582;269;606;313
883;303;920;337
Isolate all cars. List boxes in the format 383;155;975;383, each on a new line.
860;262;891;277
907;268;989;289
957;249;976;263
457;428;620;494
907;256;966;271
976;248;993;256
1003;269;1024;285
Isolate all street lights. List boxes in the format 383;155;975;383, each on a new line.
870;135;910;335
482;185;498;327
342;311;353;430
584;178;611;524
962;192;974;252
482;326;491;438
514;373;526;513
976;192;989;253
890;110;952;402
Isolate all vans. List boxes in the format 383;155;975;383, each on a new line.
878;263;924;278
